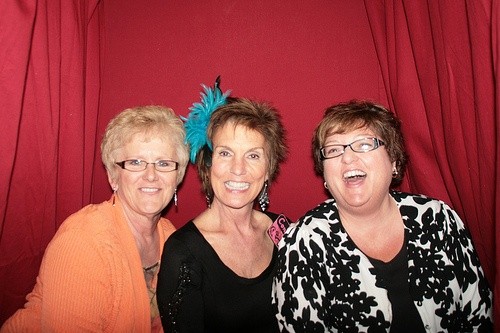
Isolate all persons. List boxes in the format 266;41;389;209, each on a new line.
156;75;293;333
0;105;189;333
271;99;493;333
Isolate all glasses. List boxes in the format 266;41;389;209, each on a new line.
320;137;384;159
116;159;178;172
